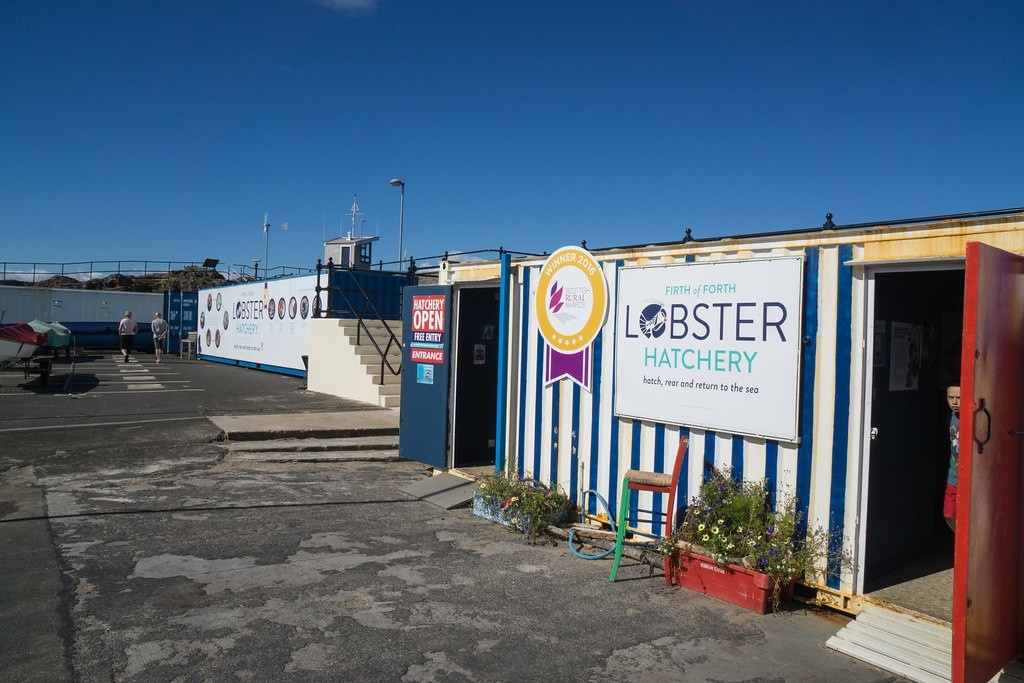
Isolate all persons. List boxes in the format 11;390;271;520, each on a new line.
943;379;961;534
151;312;167;361
119;310;138;362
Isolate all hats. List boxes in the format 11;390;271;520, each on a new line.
125;311;132;317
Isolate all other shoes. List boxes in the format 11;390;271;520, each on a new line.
125;355;129;362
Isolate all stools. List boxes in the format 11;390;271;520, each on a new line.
181;331;198;360
609;434;689;586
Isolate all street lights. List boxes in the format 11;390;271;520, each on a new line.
388;177;405;269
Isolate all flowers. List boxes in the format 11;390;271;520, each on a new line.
641;460;860;614
472;460;580;547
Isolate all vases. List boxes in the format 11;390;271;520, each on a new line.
674;549;801;614
472;491;567;532
